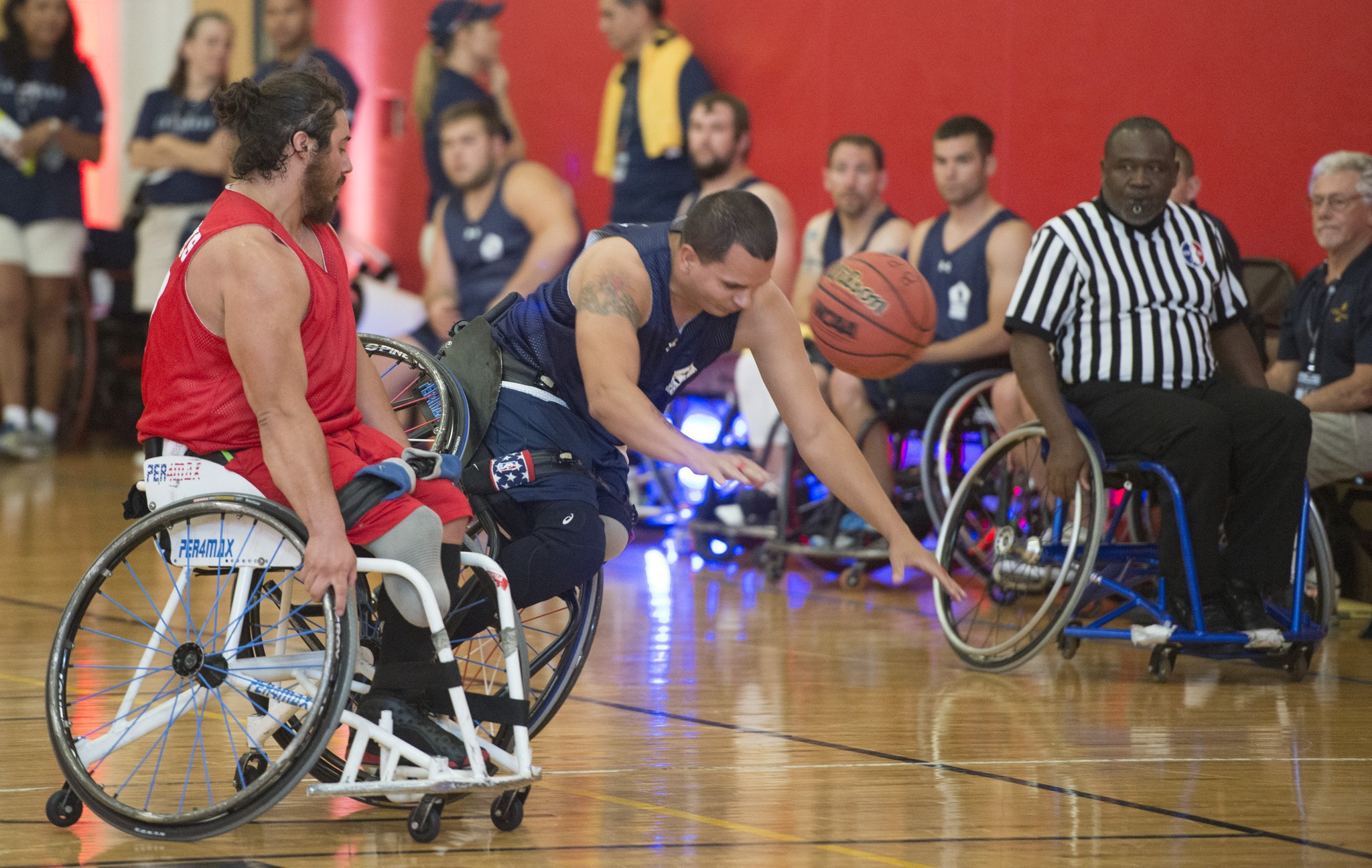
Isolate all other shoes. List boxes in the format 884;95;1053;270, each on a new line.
0;425;51;459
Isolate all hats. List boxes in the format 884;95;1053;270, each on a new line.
430;0;502;45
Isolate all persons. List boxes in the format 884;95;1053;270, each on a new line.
429;188;970;654
120;0;363;471
0;0;114;466
368;0;715;432
678;89;1372;653
127;44;489;773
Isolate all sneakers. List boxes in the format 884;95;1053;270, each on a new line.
345;693;489;768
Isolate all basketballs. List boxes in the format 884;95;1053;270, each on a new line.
806;252;937;381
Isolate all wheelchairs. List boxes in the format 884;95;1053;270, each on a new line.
931;411;1339;682
228;330;608;807
43;435;543;842
679;370;1133;599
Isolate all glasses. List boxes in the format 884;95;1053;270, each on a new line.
1308;194;1372;213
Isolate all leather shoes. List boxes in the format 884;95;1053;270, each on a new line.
1223;593;1272;654
1166;597;1241;654
